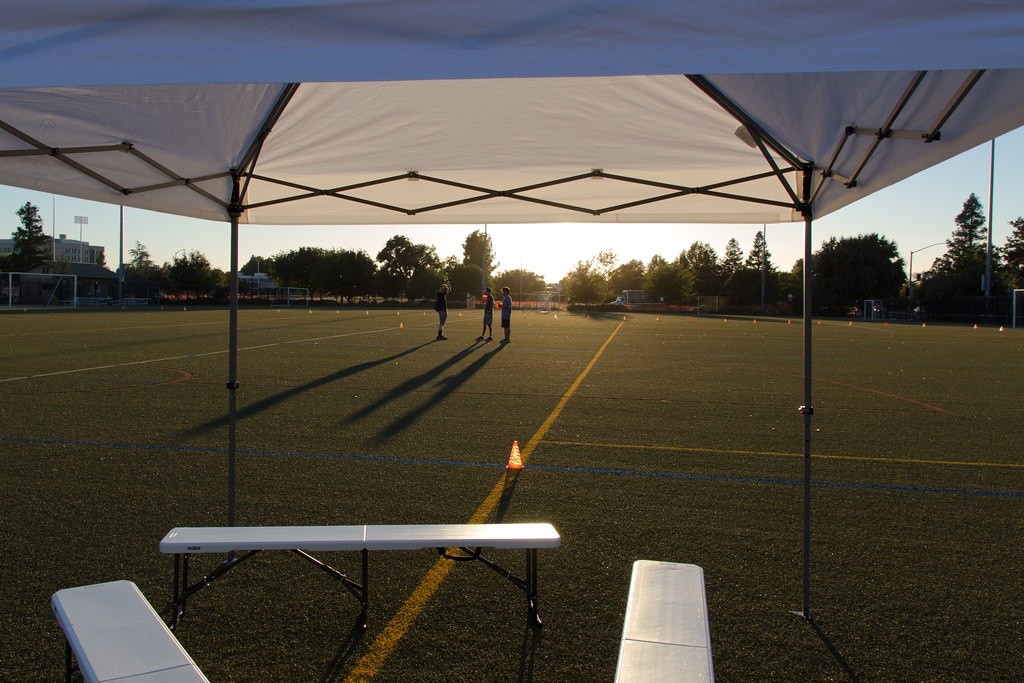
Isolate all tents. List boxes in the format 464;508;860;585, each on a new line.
1;0;1024;621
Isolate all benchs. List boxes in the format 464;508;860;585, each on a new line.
158;522;562;635
614;560;715;683
50;580;211;683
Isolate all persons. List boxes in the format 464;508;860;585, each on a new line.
475;287;494;341
501;287;512;343
435;284;451;340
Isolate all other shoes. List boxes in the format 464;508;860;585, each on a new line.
476;336;484;340
484;337;493;341
499;339;510;343
437;336;448;340
